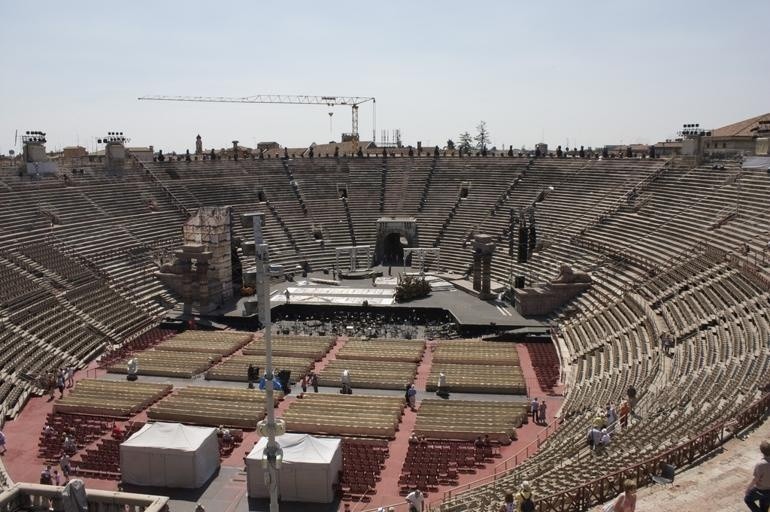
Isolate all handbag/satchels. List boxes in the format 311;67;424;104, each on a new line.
586;430;593;446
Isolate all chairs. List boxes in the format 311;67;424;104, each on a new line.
398;441;492;497
339;441;390;503
36;412;141;481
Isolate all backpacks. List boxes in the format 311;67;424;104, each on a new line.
521;492;535;512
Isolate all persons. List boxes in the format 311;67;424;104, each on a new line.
651;459;675;489
404;489;425;512
744;439;770;512
612;478;637;512
743;243;751;256
493;480;535;512
408;432;428;447
112;424;122;440
40;417;94;486
0;431;8;456
217;425;232;448
529;398;547;427
247;364;255;389
301;371;320;392
376;506;395;512
474;433;491;448
659;329;674;355
404;381;416;410
45;364;75;400
586;385;637;454
47;497;55;512
284;289;291;305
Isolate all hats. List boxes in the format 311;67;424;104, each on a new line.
592;416;603;427
520;480;531;492
